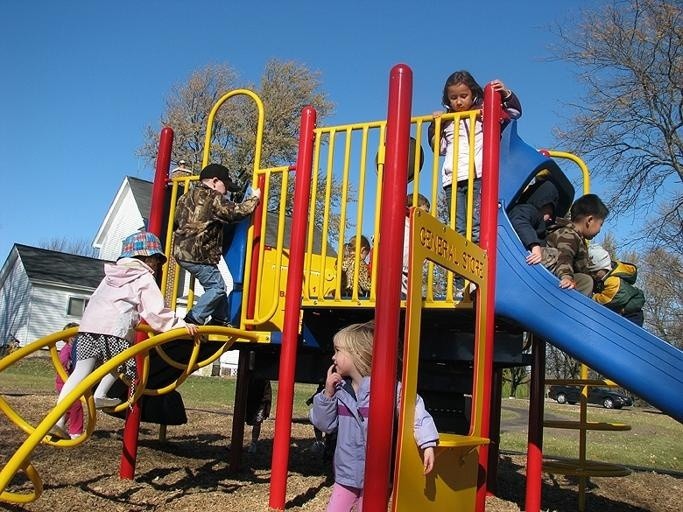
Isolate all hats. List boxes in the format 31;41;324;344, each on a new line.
587;245;613;273
200;164;242;192
117;233;167;264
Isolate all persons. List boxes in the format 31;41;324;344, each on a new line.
47;229;200;440
306;318;440;512
54;322;84;440
170;163;261;328
506;178;560;267
586;242;644;327
244;379;272;454
340;234;371;297
426;69;520;303
399;192;432;302
540;193;609;299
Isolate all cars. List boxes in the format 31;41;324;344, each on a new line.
546;384;634;409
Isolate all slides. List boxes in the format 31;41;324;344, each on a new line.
496;200;682;423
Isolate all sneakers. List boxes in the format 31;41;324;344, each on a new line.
94;398;122;409
182;311;201;325
49;423;83;439
209;320;233;328
